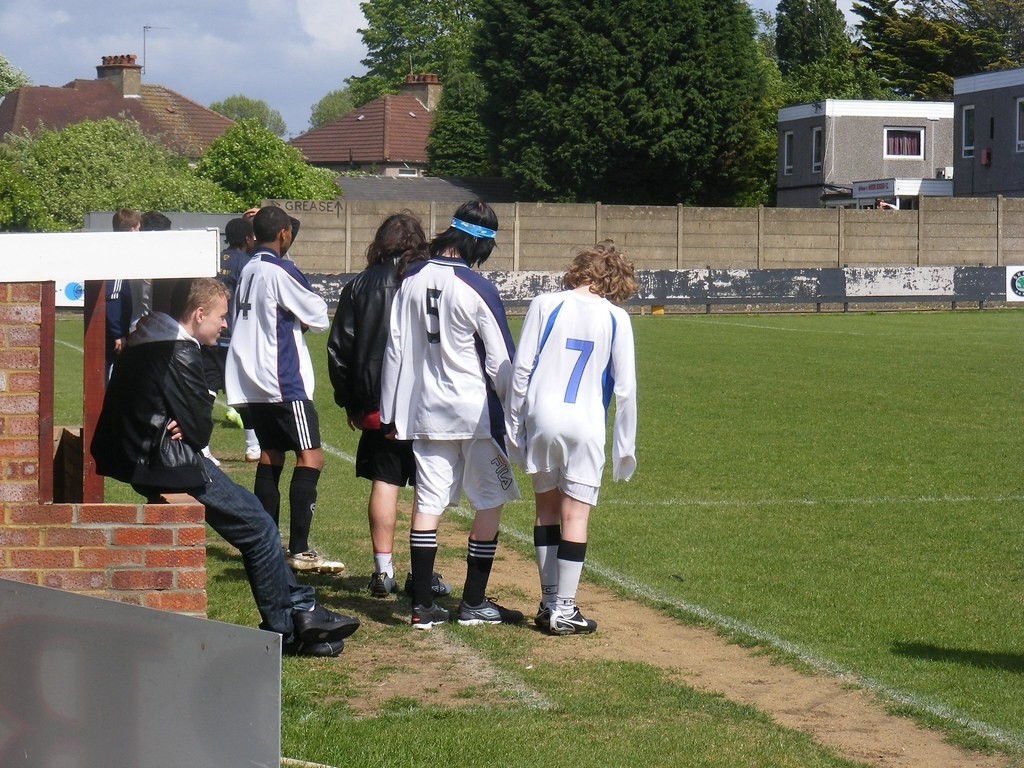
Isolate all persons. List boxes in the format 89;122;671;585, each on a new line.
97;208;346;573
875;199;885;210
90;279;361;659
504;240;637;633
328;200;453;596
378;201;515;629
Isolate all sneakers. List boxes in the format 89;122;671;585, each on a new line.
204;453;220;467
534;601;558;628
293;604;361;644
282;632;344;657
367;573;399;598
550;606;597;637
284;548;345;574
411;602;456;630
404;572;451;597
245;447;261;462
225;409;244;430
456;596;524;626
